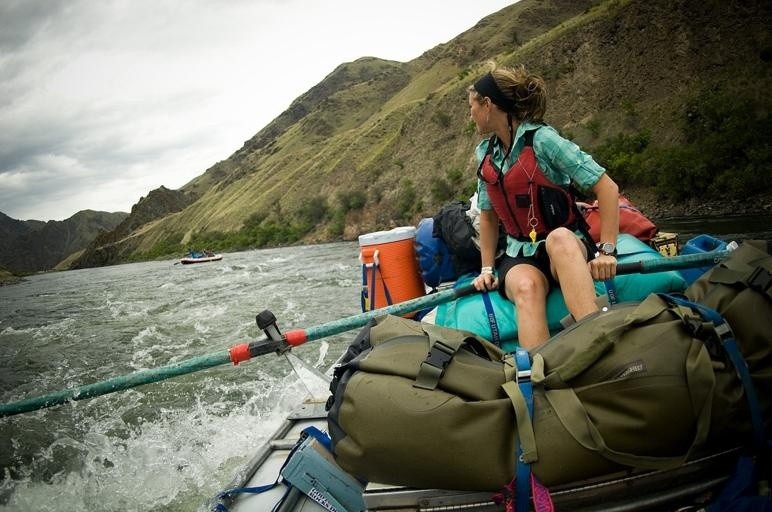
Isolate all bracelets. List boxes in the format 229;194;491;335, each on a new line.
479;266;494;273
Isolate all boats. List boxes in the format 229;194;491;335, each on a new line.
180;254;222;265
208;322;771;510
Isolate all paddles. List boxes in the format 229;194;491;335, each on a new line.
173;261;180;265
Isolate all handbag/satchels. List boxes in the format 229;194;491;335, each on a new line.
432;198;482;276
326;241;772;490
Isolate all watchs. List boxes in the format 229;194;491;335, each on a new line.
597;242;618;257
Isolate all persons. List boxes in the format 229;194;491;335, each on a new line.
204;248;215;257
465;62;620;353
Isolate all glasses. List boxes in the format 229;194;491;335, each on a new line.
476;166;501;186
